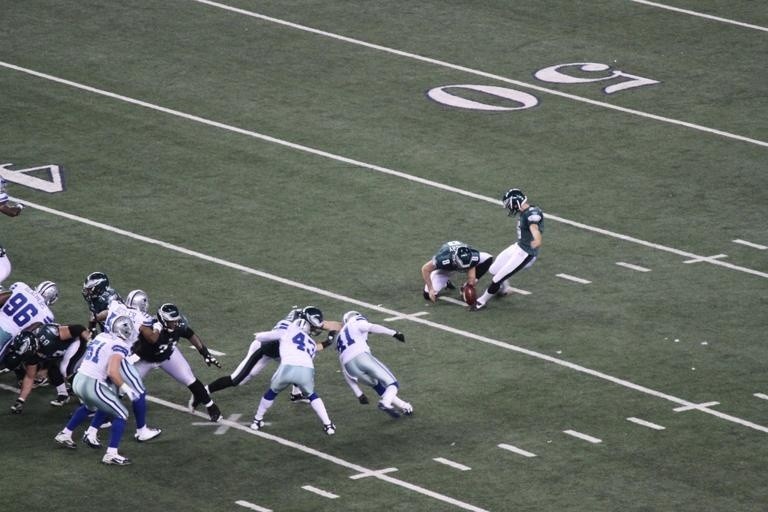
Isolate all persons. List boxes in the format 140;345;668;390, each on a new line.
189;307;414;434
1;272;224;465
0;176;25;292
421;239;509;303
470;188;547;311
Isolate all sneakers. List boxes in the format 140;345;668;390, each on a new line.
323;422;338;437
289;393;312;405
378;397;414;422
252;416;266;431
443;277;510;309
31;375;161;465
188;387;223;423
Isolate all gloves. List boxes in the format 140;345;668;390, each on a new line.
393;332;405;340
10;400;24;415
355;394;368;405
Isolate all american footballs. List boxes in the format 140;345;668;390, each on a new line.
463;284;476;305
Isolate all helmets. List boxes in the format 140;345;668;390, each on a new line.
81;271;180;339
291;306;364;338
452;247;472;269
502;188;527;218
35;279;60;306
9;323;58;359
